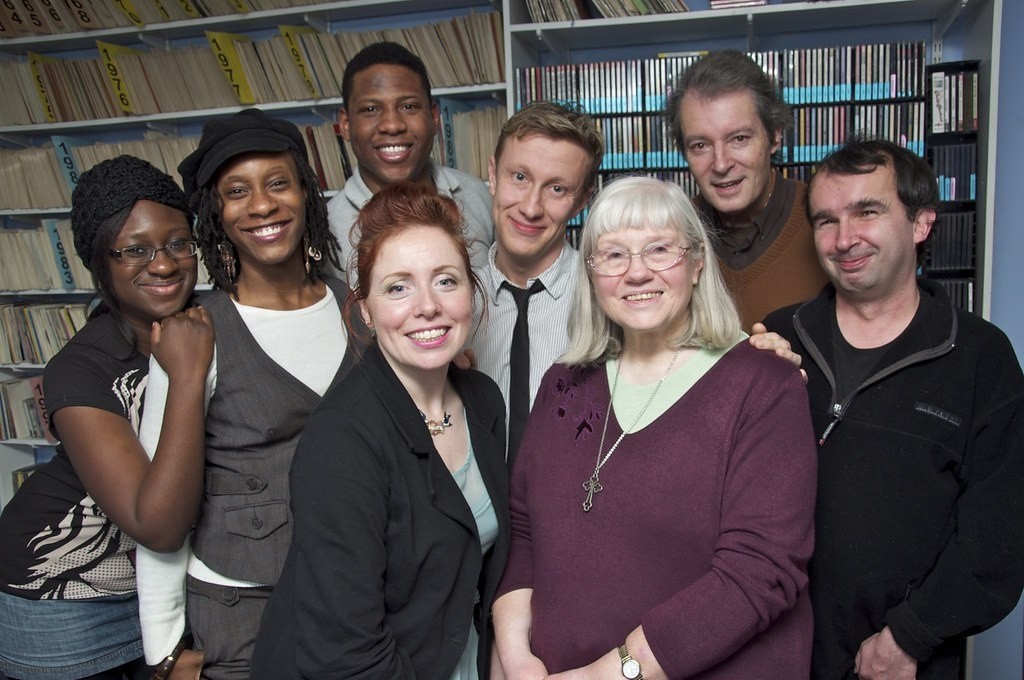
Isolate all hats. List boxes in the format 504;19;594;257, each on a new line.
69;155;195;268
177;108;308;186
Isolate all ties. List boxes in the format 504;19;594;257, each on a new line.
501;278;546;476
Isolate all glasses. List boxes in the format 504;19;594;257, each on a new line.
107;235;197;264
586;242;694;277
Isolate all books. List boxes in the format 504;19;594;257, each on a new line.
510;0;980;315
0;0;506;493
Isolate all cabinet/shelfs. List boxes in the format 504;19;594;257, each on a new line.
503;2;1003;323
0;1;512;526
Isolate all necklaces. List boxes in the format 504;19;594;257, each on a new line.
421;408;451;436
581;341;685;512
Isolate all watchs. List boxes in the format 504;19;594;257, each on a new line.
618;644;644;680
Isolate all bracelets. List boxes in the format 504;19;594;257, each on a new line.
151;641;185;680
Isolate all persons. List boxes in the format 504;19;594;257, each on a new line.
487;175;814;680
0;155;214;680
667;46;832;331
134;108;479;680
461;98;811;467
245;179;510;680
318;40;496;317
749;137;1024;680
